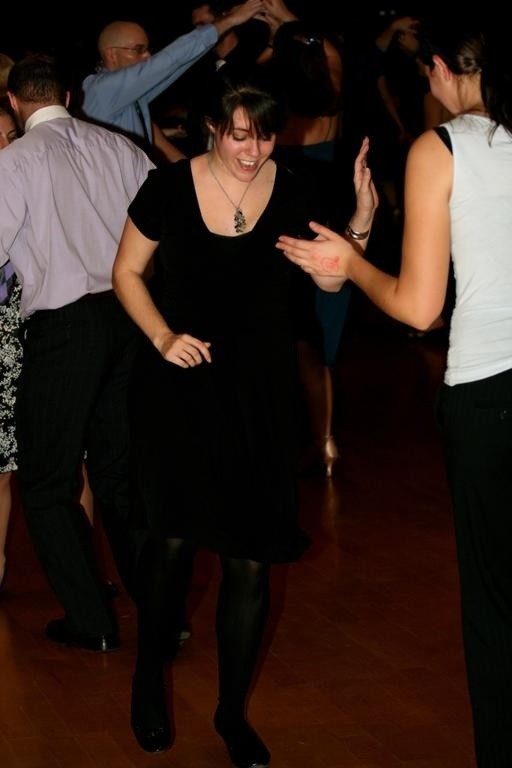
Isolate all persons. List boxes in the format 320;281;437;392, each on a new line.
85;1;265;144
156;0;345;474
1;100;95;575
2;53;197;651
365;17;446;340
104;57;384;767
270;1;509;768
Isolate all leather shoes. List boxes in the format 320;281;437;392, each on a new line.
129;660;174;752
46;617;121;655
213;707;270;768
